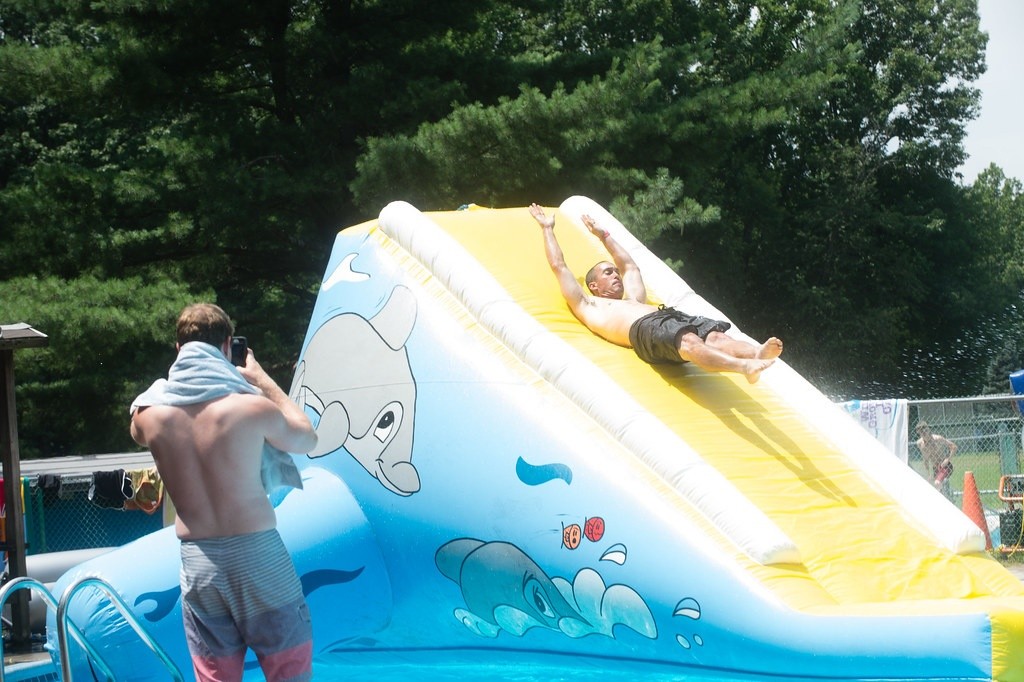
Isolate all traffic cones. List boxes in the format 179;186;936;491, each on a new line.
963;471;992;552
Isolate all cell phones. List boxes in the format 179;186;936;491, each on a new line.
230;337;247;368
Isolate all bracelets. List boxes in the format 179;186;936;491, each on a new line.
599;232;610;241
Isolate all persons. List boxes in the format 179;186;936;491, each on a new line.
916;420;957;501
129;301;318;682
528;201;783;384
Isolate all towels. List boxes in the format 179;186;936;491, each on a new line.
129;341;303;497
